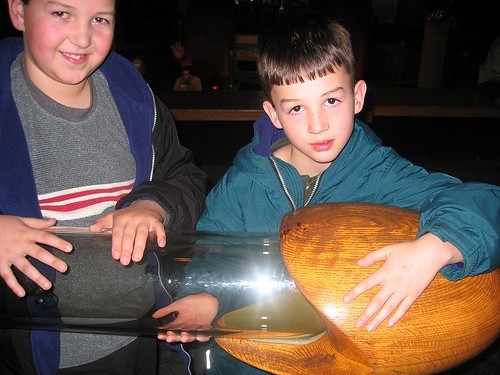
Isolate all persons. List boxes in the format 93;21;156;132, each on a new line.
123;39;233;93
0;0;206;375
151;18;500;375
